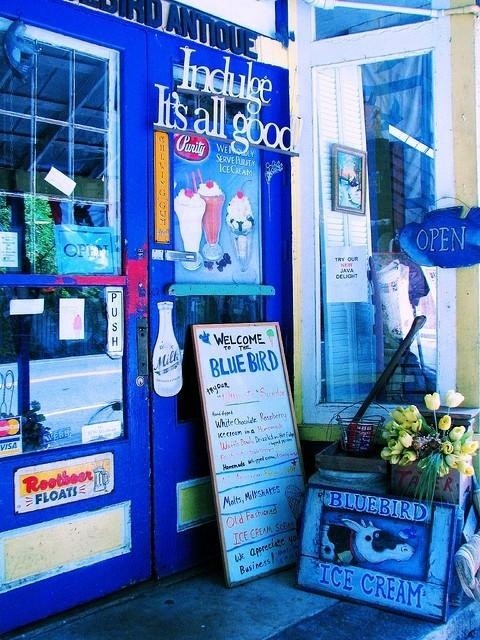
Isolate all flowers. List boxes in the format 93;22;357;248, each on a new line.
381;388;479;509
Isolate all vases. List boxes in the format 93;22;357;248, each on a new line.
386;460;471;506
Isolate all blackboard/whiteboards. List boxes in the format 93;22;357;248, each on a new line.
192;322;306;588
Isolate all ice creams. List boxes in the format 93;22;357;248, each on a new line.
226;179;257;272
173;171;206;262
197;168;225;246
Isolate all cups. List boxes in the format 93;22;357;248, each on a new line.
92;466;111;493
173;193;257;285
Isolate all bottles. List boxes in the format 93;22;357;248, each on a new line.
150;300;186;399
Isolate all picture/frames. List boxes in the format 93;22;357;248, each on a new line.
331;143;368;216
297;483;455;624
